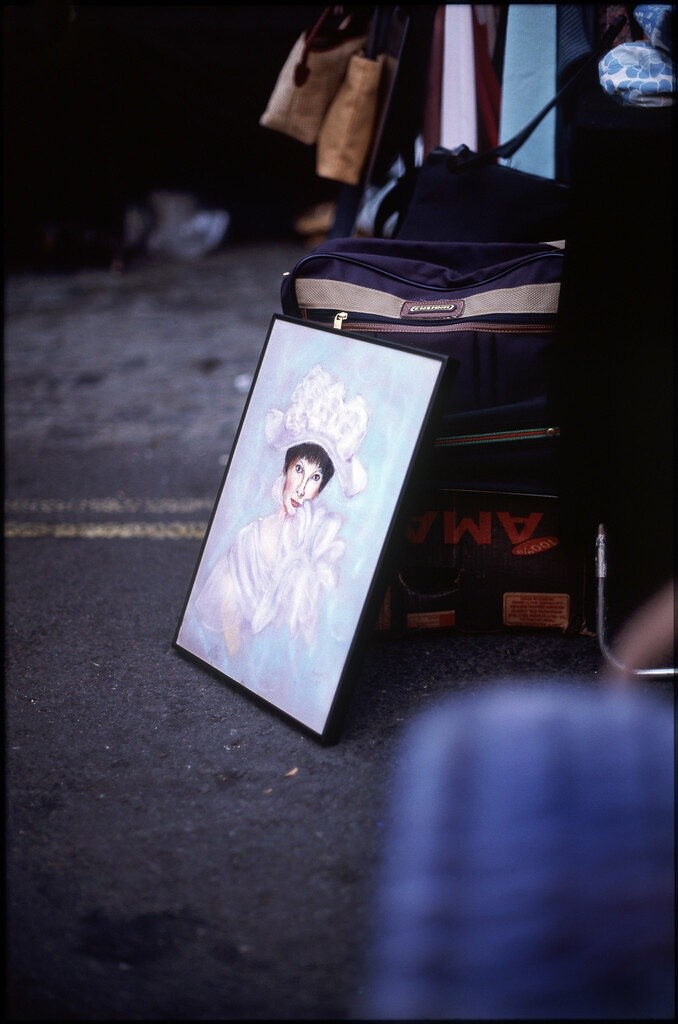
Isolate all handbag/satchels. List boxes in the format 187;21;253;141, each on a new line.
282;239;564;490
368;149;567;243
257;2;383;184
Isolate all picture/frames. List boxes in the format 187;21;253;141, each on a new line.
170;314;459;745
